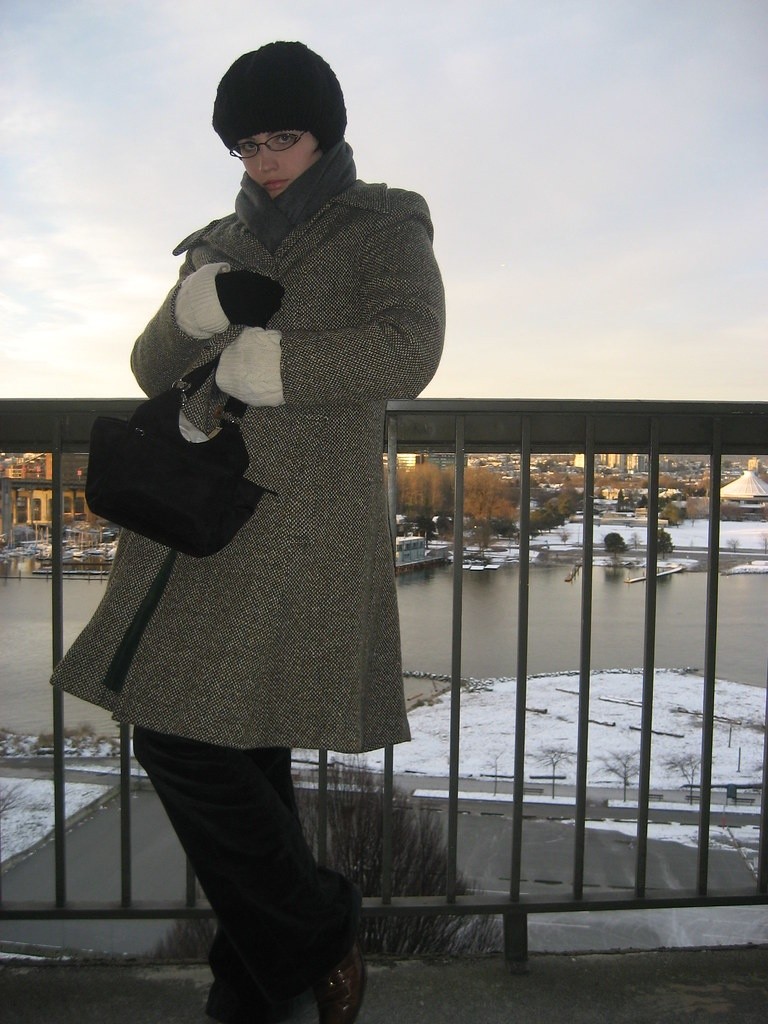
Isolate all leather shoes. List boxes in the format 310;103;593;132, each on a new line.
313;886;366;1024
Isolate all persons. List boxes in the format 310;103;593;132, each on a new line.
47;42;445;1022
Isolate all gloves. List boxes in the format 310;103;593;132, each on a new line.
215;268;285;325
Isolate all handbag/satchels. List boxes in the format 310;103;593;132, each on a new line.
85;387;267;559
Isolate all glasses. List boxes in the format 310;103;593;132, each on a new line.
230;129;308;158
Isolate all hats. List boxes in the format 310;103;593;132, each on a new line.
212;42;347;153
